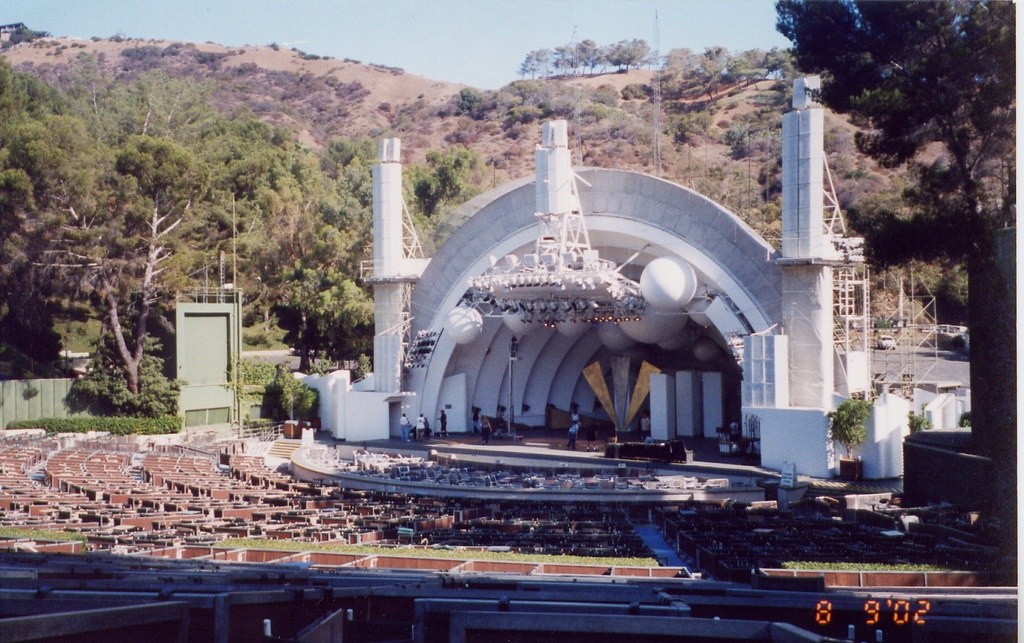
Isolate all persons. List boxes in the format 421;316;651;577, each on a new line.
729;417;740;441
399;404;507;443
565;409;650;450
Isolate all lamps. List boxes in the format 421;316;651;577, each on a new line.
471;273;645;328
404;329;437;369
727;328;750;366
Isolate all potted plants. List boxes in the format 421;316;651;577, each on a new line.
826;398;874;477
277;371;322;439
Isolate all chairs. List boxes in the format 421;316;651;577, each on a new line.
671;439;693;464
